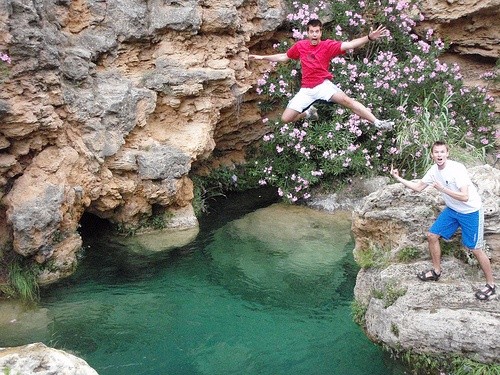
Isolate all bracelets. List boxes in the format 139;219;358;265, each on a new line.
367;35;373;41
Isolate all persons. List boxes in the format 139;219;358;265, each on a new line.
248;19;395;133
390;140;496;300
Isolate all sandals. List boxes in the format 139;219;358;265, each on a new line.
475;283;495;300
417;269;441;281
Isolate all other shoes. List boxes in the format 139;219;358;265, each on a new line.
308;105;318;121
375;119;395;132
